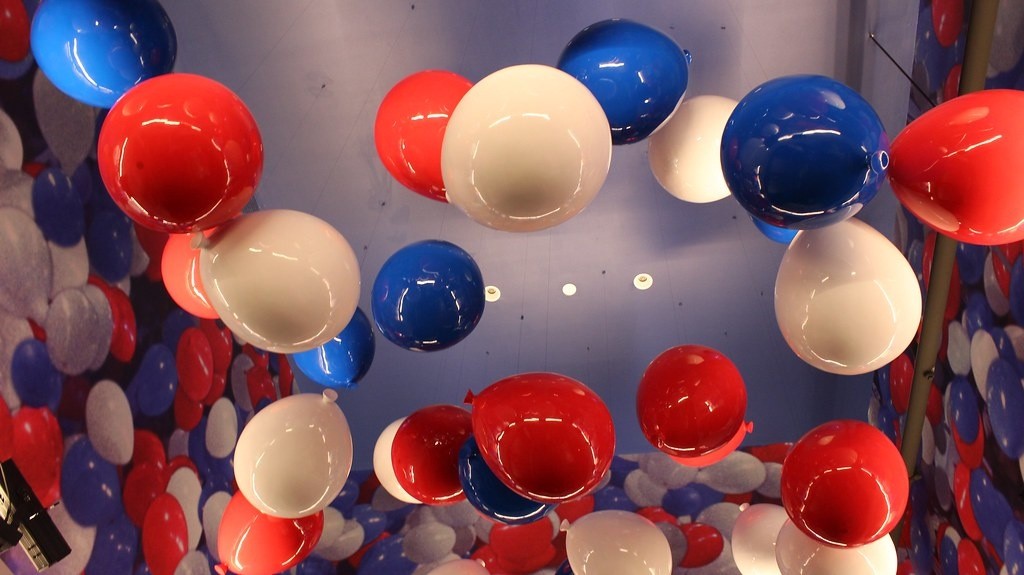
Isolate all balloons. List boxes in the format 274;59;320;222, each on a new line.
201;209;362;353
234;389;352;518
750;215;803;243
885;89;1024;246
556;20;691;145
391;404;476;507
780;420;910;549
214;491;321;575
372;240;485;353
646;94;752;203
161;228;223;320
560;510;673;575
636;344;747;458
98;72;263;233
668;421;755;467
773;218;922;376
731;502;788;575
294;306;375;389
464;374;617;505
459;435;559;523
28;0;177;107
775;518;900;575
1;0;1024;575
372;418;426;504
441;63;612;233
374;71;474;200
722;76;891;231
